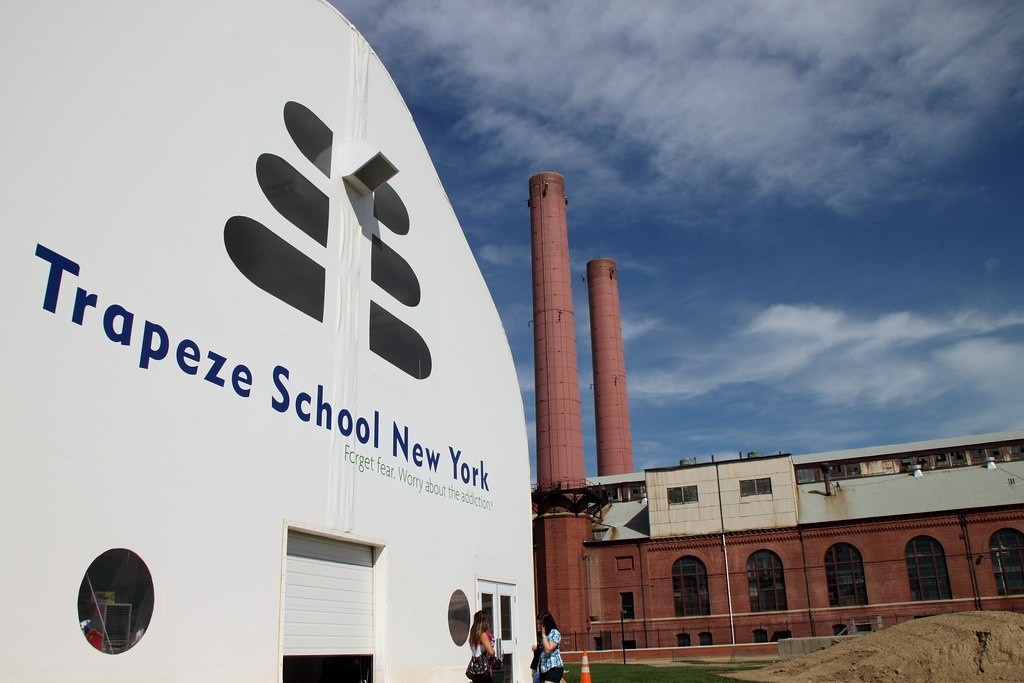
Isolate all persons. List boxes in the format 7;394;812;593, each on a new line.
531;611;564;683
466;611;495;683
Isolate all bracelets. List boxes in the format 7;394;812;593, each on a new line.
542;636;547;638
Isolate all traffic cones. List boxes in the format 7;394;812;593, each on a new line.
580;649;592;683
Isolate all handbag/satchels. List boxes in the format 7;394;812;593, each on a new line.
465;650;490;679
